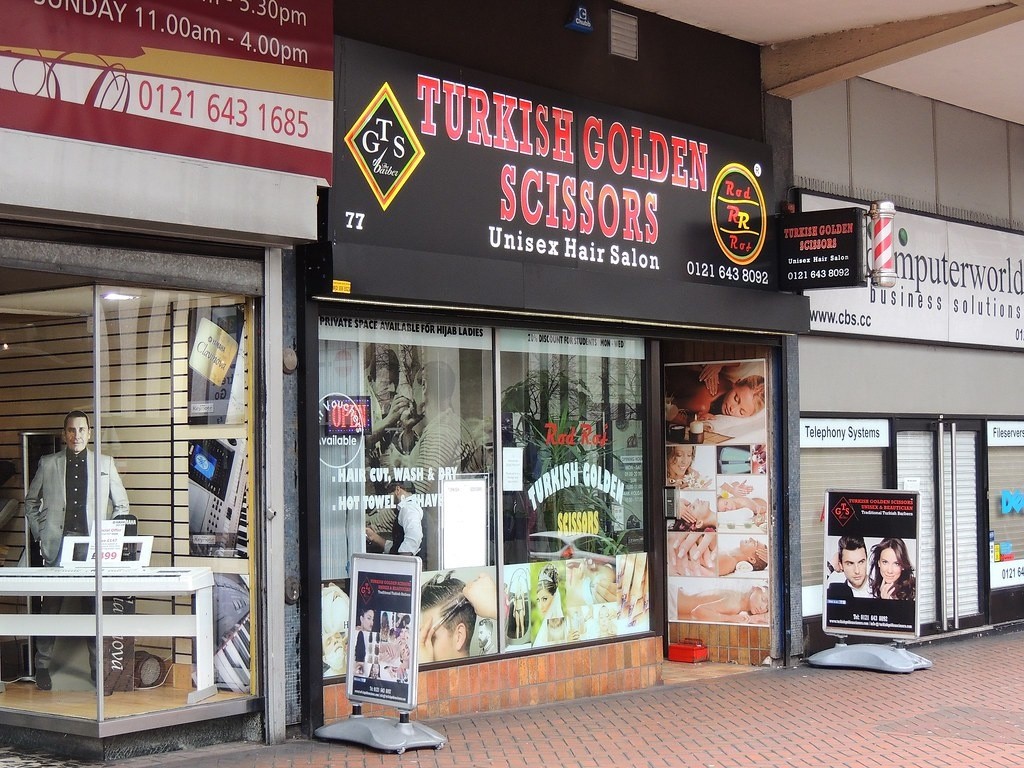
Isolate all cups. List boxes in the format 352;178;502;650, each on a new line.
670;424;686;443
689;429;704;444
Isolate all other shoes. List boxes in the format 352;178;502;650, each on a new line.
36;668;52;690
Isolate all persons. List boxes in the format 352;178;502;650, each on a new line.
354;609;411;685
827;537;879;598
668;580;768;624
504;582;525;647
477;619;497;655
672;498;717;532
532;563;567;648
420;570;476;662
23;410;131;690
666;446;713;490
566;553;649;643
719;536;768;575
665;366;765;431
717;476;767;526
419;572;497;664
361;478;428;572
668;533;717;577
868;538;916;601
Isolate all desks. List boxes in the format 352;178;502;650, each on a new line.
0;566;217;704
